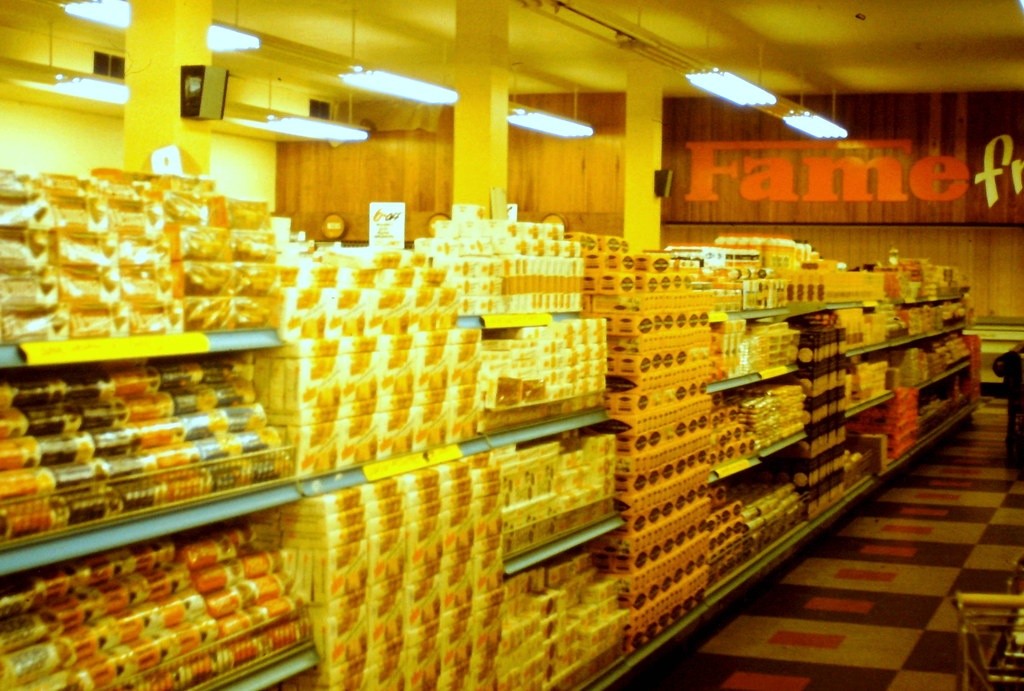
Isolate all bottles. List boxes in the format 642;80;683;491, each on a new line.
712;231;819;271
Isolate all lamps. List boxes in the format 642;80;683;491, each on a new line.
0;57;367;143
519;0;851;139
70;0;597;140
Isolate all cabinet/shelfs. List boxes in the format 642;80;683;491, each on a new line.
0;280;973;691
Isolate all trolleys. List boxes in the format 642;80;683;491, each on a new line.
951;591;1024;691
